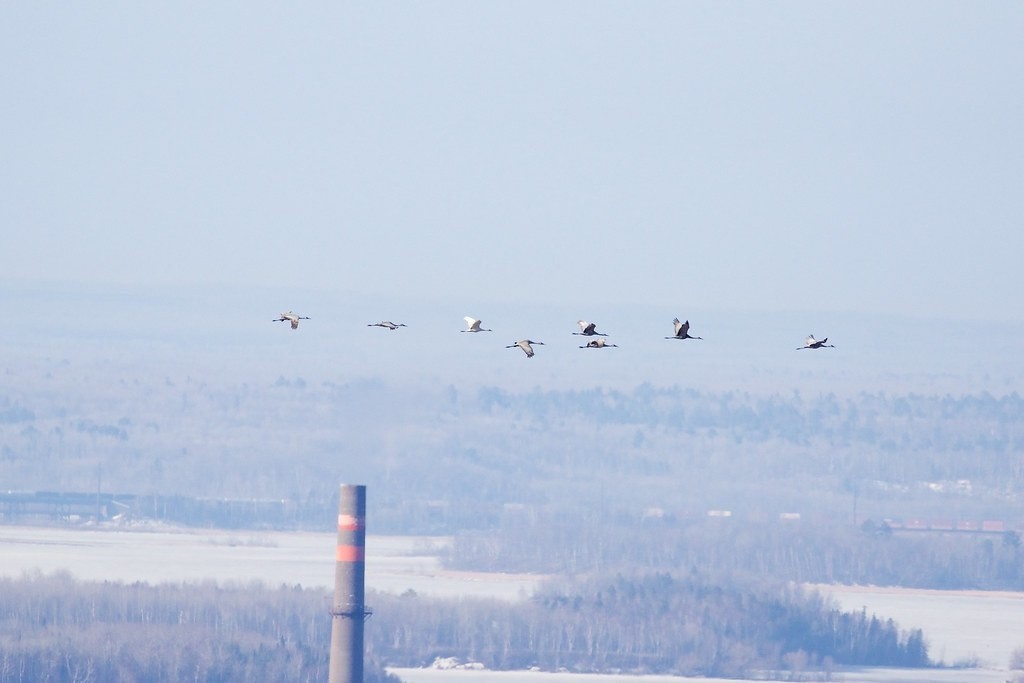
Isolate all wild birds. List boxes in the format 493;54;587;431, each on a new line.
664;317;703;340
795;334;836;351
579;337;620;349
272;309;312;330
460;316;492;333
367;320;407;331
504;337;547;359
572;319;609;337
